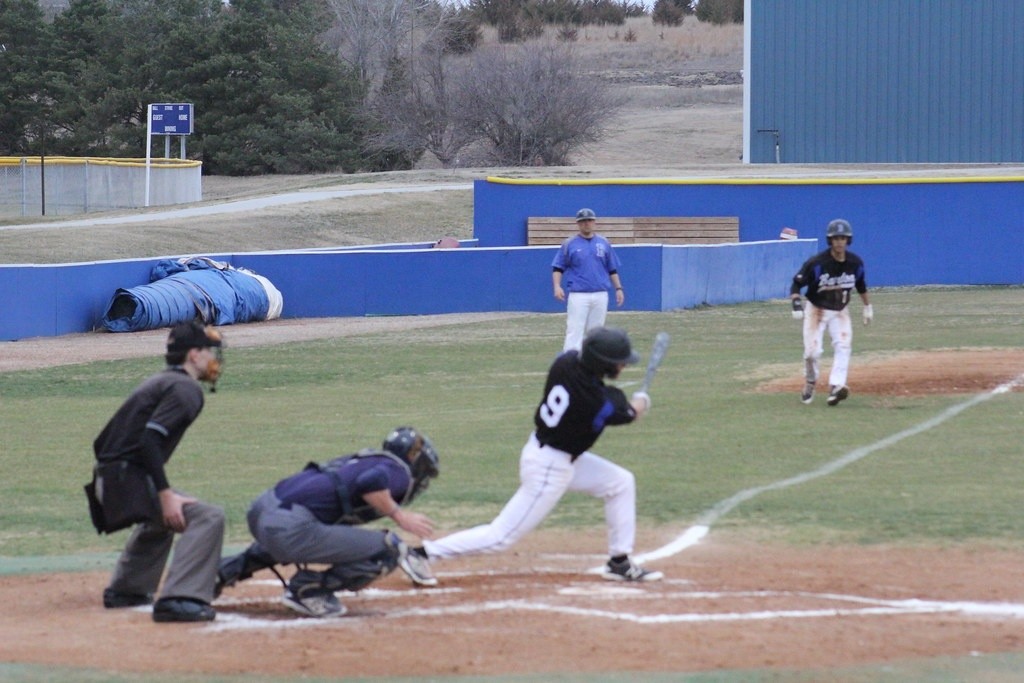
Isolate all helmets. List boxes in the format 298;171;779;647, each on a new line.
575;207;596;223
383;426;425;467
165;319;207;351
825;219;853;246
582;325;640;374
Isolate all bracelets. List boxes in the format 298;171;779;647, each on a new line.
616;287;622;290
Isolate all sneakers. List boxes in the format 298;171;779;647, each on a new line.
398;543;437;586
602;558;663;582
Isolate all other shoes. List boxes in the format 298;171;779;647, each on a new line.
826;385;848;405
281;578;350;617
103;588;154;608
153;597;216;623
800;381;816;404
212;575;223;598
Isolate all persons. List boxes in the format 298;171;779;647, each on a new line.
92;321;226;623
790;220;872;406
551;209;624;355
214;427;441;616
397;327;664;585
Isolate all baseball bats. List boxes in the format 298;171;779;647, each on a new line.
641;330;672;392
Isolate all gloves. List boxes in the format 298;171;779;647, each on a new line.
791;298;804;320
862;304;873;325
632;391;651;410
